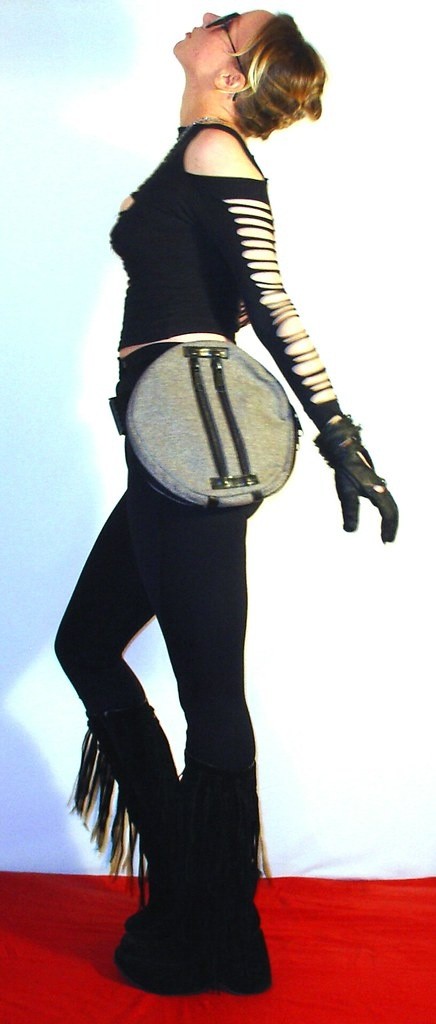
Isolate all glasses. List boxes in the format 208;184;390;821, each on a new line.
206;12;248;101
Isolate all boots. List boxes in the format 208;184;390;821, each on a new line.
67;699;272;996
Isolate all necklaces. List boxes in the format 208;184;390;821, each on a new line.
178;117;223;143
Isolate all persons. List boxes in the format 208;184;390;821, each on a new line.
54;9;400;998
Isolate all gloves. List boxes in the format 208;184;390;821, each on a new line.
313;416;398;543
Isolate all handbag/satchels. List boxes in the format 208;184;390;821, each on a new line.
109;340;302;510
